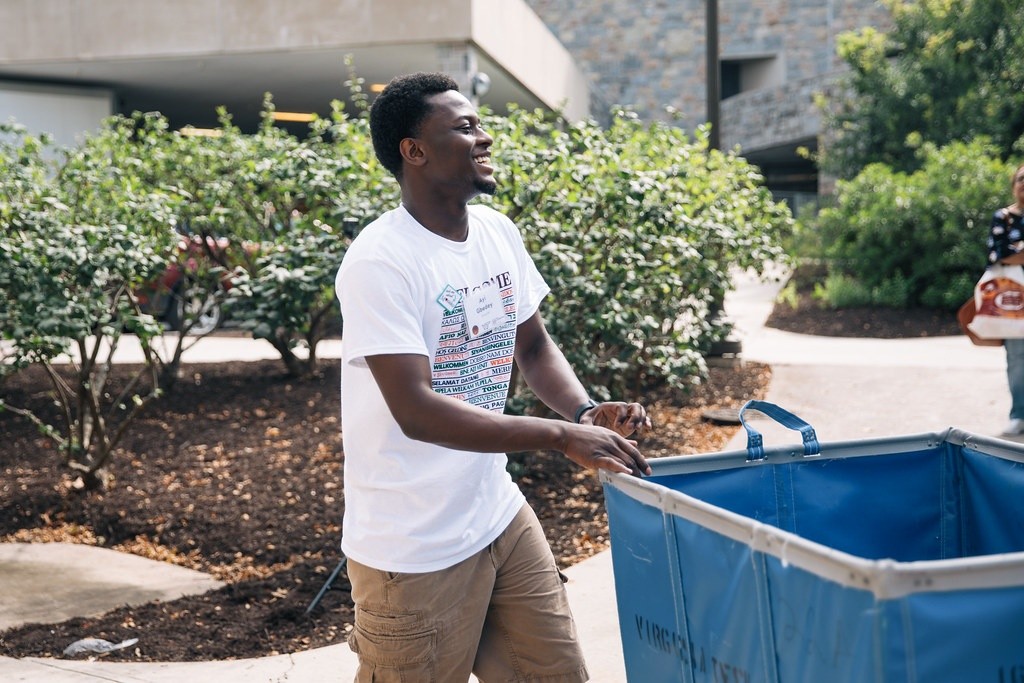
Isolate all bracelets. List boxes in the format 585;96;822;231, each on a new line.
573;398;597;423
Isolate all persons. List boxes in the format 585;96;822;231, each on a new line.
333;72;652;683
984;163;1024;437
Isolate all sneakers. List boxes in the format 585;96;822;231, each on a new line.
1003;419;1024;434
